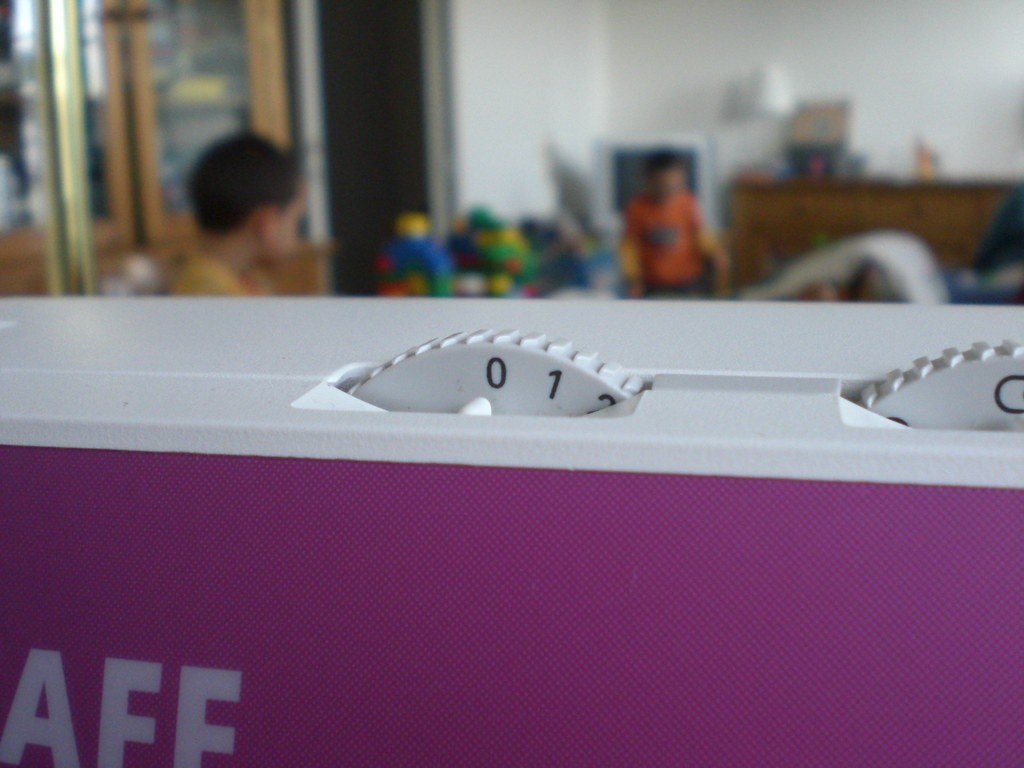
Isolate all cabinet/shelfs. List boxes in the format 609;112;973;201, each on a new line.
0;1;340;297
730;181;1020;301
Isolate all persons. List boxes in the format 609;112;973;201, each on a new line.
169;131;325;296
619;150;732;303
